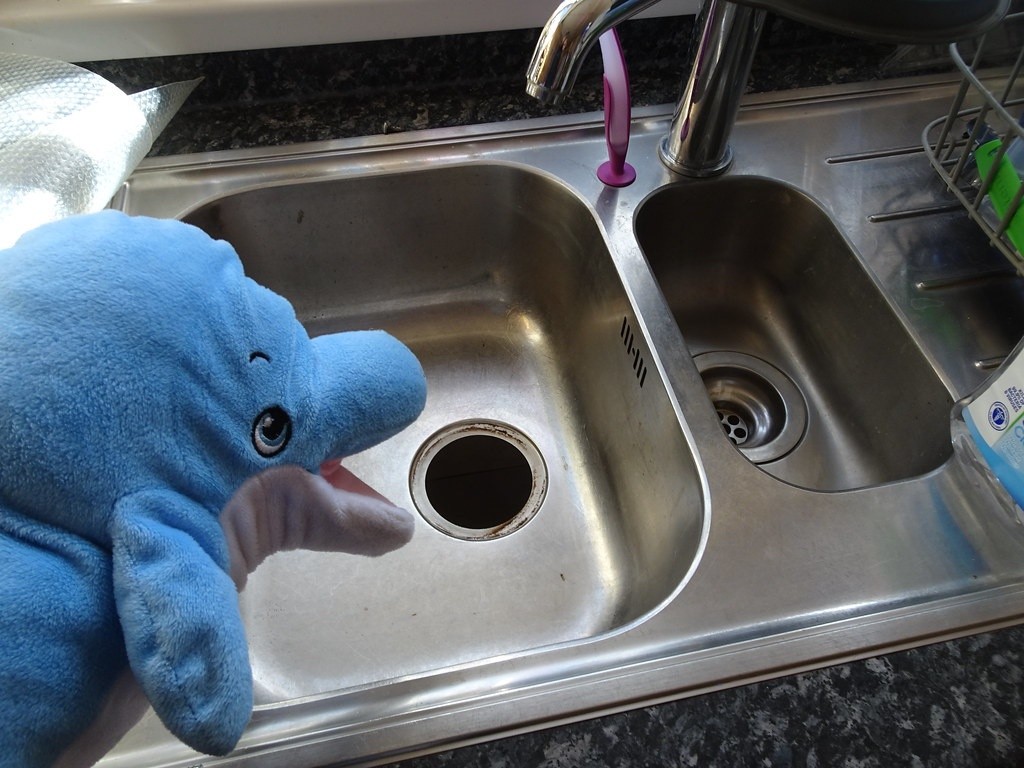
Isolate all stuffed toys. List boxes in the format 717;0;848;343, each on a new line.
0;208;427;768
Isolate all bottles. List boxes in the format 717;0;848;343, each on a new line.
948;330;1024;538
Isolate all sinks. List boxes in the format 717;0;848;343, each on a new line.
623;137;972;708
63;141;715;768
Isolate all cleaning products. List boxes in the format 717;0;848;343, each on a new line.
946;334;1024;543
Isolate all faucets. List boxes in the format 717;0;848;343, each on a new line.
520;0;675;115
